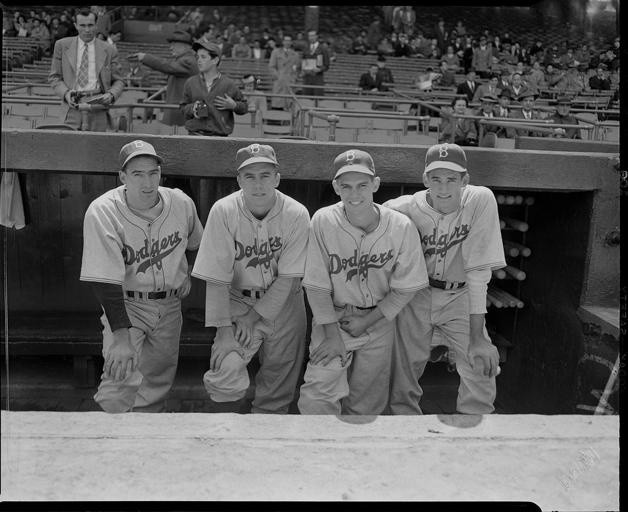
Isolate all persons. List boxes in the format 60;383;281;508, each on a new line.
269;35;301;125
376;57;395;90
413;58;456;92
504;55;622;92
473;94;508;139
130;32;199;126
320;5;619;56
300;30;330;98
191;145;310;414
438;96;477;147
492;89;514;117
180;40;249;137
455;68;481;102
300;150;429;414
476;74;503;100
81;141;204;412
440;56;504;73
380;143;506;414
545;96;583;139
91;5;282;54
50;8;128;133
358;65;387;92
507;88;549;137
284;5;300;52
2;7;79;42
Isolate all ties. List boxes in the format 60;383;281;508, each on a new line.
77;46;88;88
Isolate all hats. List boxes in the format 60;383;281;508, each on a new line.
236;143;280;170
481;93;499;103
555;96;573;105
518;90;540;102
192;41;222;59
118;139;164;170
332;150;377;178
424;143;467;174
497;89;515;101
167;32;193;46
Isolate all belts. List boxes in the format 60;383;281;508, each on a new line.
244;289;265;298
127;291;180;299
358;306;379;310
429;278;466;290
